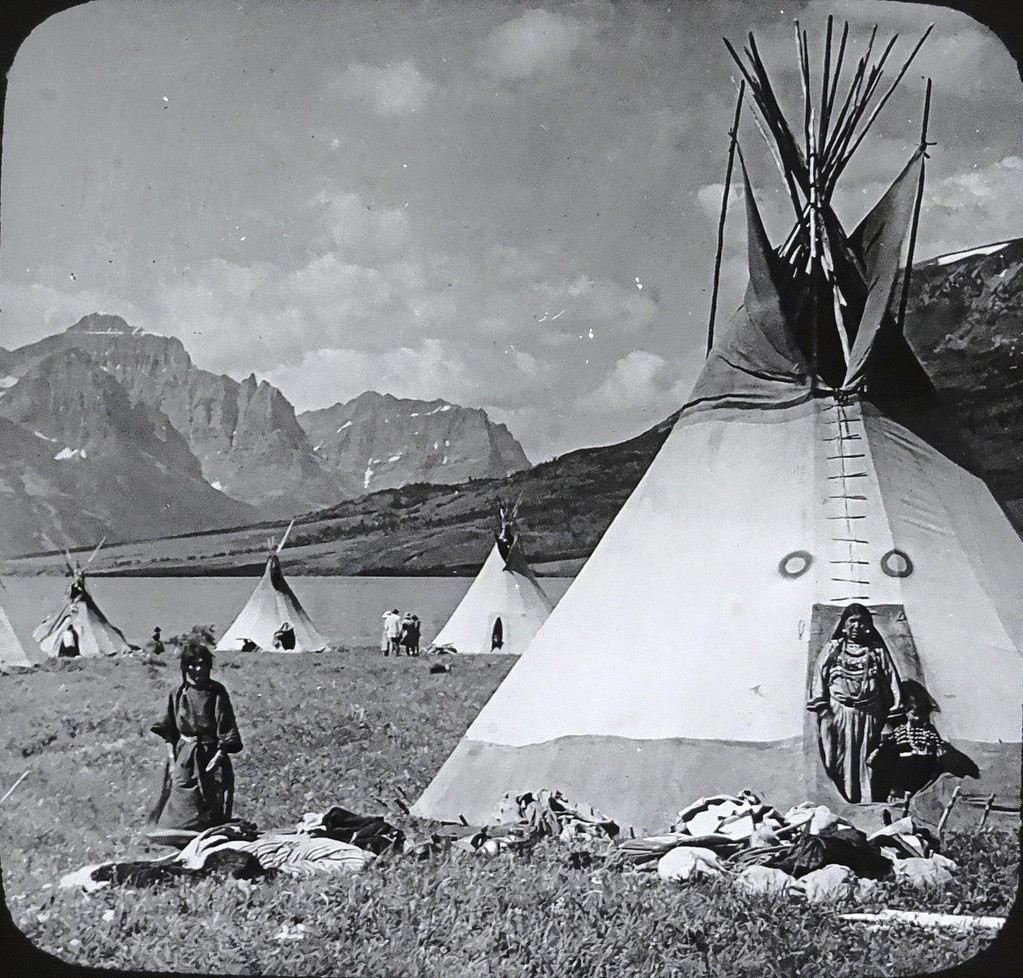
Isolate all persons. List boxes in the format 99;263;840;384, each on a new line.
380;609;421;657
151;644;243;831
807;602;945;805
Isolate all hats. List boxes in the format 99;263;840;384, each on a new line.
154;627;161;631
403;612;412;618
392;609;399;613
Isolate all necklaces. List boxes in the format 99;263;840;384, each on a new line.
842;639;871;699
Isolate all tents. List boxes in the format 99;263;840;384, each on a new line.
216;520;331;653
427;494;552;656
401;17;1022;851
33;537;133;661
0;604;39;672
